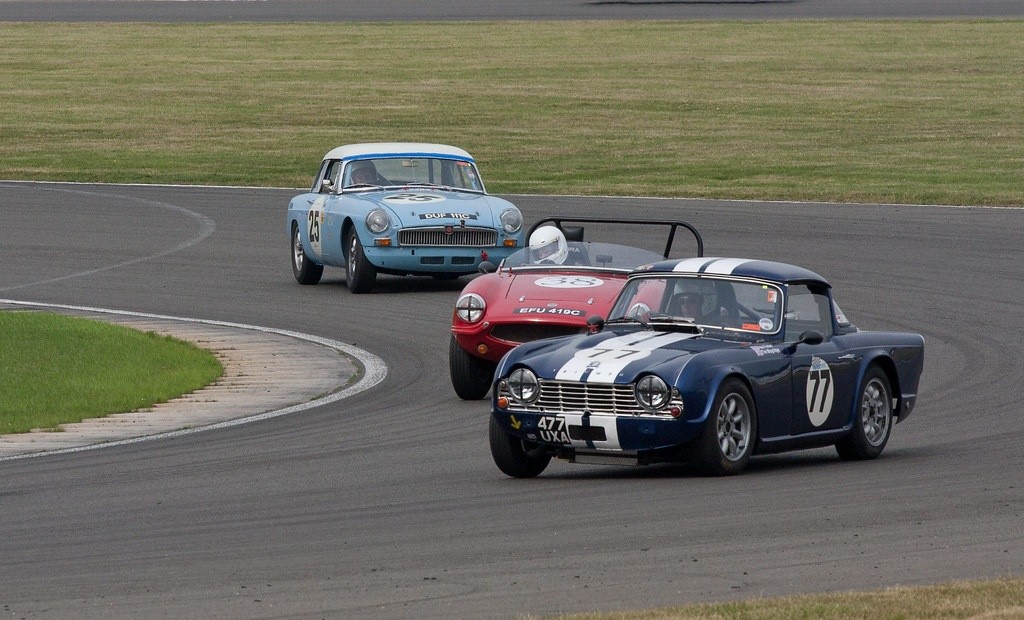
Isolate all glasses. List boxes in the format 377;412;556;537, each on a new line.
678;296;700;305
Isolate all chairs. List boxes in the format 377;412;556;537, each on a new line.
561;226;583;257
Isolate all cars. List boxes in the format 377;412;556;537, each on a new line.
484;255;929;480
451;216;707;399
286;140;523;295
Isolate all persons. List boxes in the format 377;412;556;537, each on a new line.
638;278;722;329
347;159;380;190
527;225;575;268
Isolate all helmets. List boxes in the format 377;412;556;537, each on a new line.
673;279;718;317
350;160;377;185
529;226;569;265
